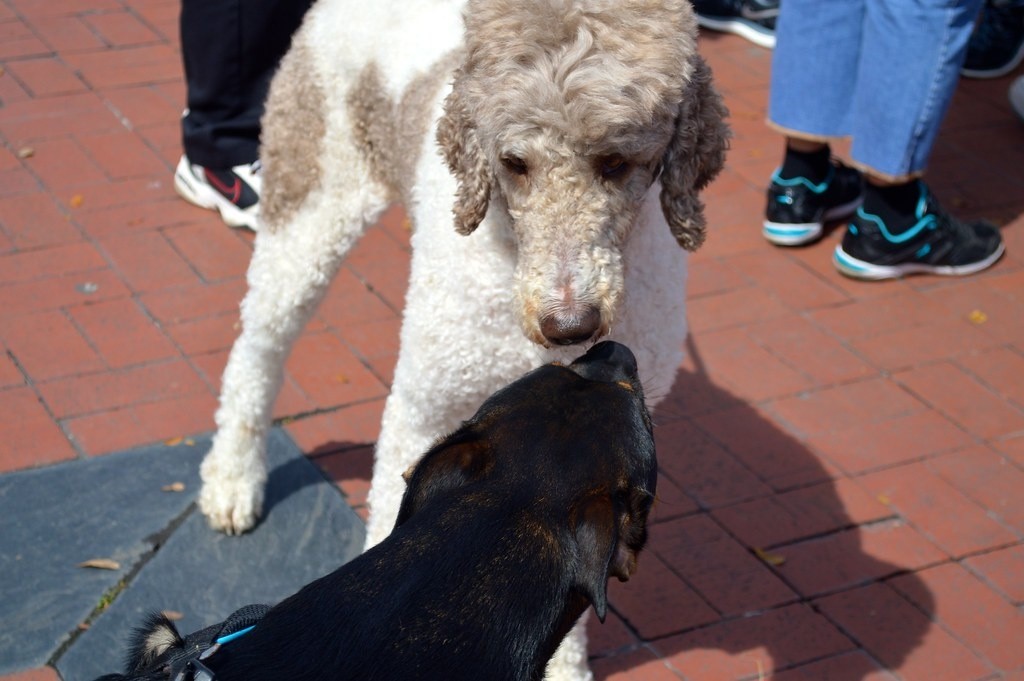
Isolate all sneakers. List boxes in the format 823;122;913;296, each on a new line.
689;0;780;47
761;155;870;245
832;178;1006;280
173;149;267;234
960;0;1024;79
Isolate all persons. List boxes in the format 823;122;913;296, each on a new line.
174;0;324;236
690;0;1024;282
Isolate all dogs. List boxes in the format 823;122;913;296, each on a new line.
194;2;730;679
71;341;659;681
